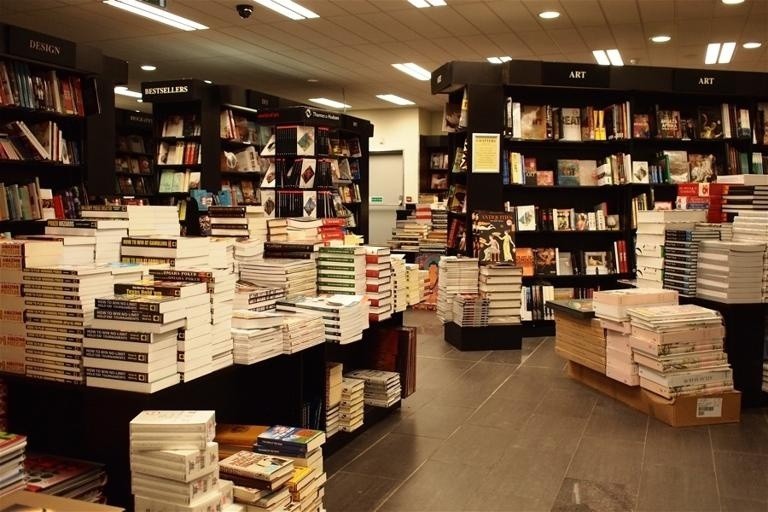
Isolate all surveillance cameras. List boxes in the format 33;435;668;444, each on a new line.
237;5;254;18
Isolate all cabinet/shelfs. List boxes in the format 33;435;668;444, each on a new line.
419;58;768;339
0;21;374;250
